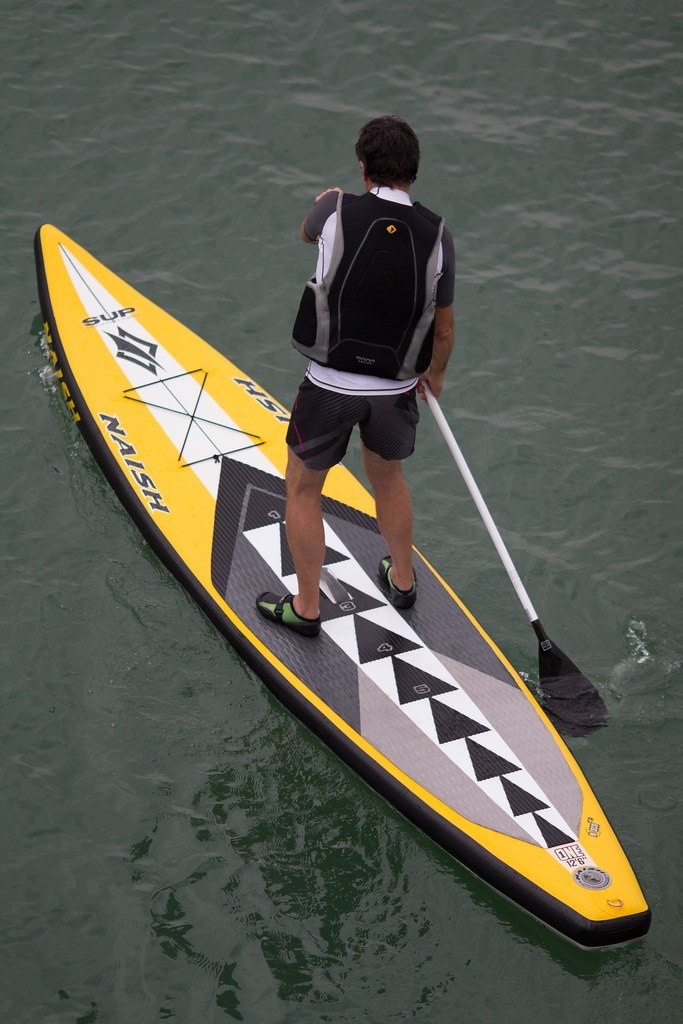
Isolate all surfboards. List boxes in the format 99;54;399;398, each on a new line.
36;220;655;950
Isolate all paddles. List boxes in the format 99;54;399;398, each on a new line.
421;379;612;730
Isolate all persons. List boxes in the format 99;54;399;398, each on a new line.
256;116;455;637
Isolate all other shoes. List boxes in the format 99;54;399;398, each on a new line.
256;591;321;638
379;555;418;609
